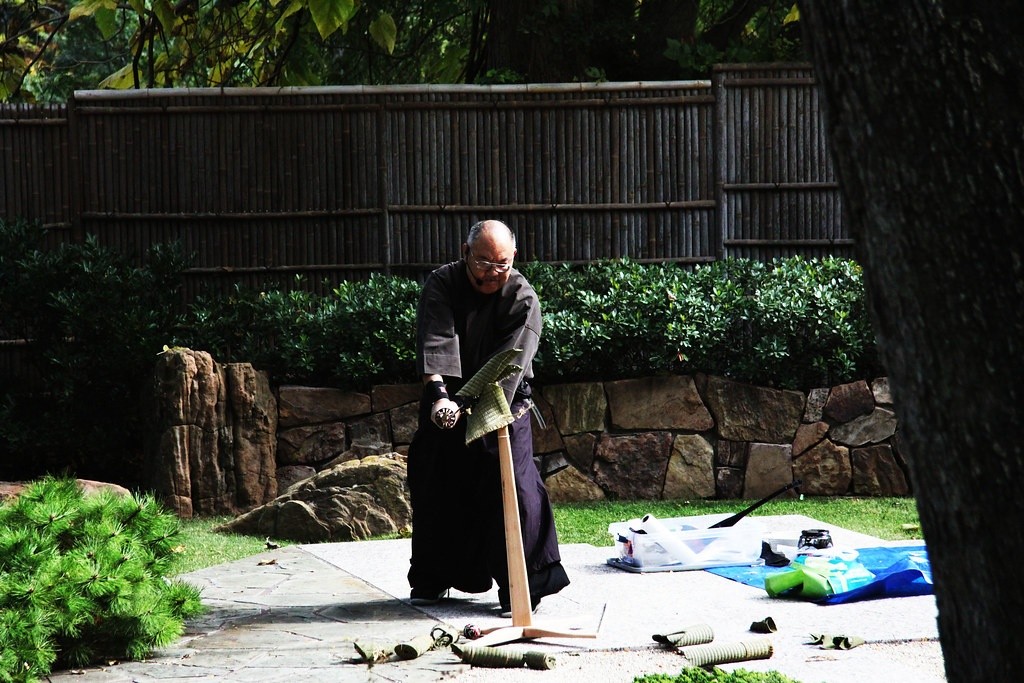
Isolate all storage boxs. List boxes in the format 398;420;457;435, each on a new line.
608;512;763;567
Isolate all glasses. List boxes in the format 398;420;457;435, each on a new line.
466;242;515;273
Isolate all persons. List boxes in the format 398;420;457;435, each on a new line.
406;220;570;620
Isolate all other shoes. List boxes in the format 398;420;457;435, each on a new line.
409;587;447;604
501;598;541;617
